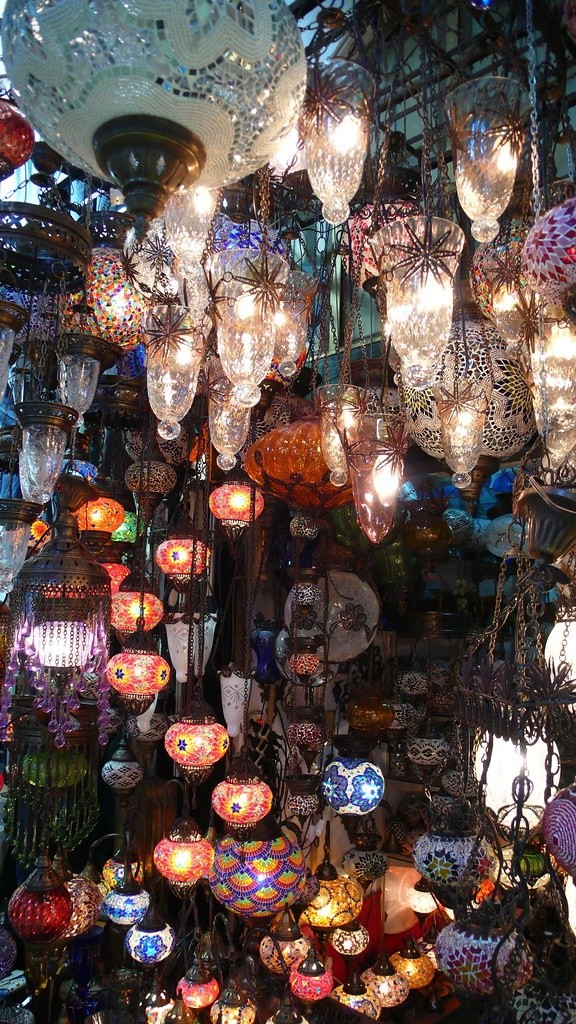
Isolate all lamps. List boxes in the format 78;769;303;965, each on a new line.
0;0;575;1024
